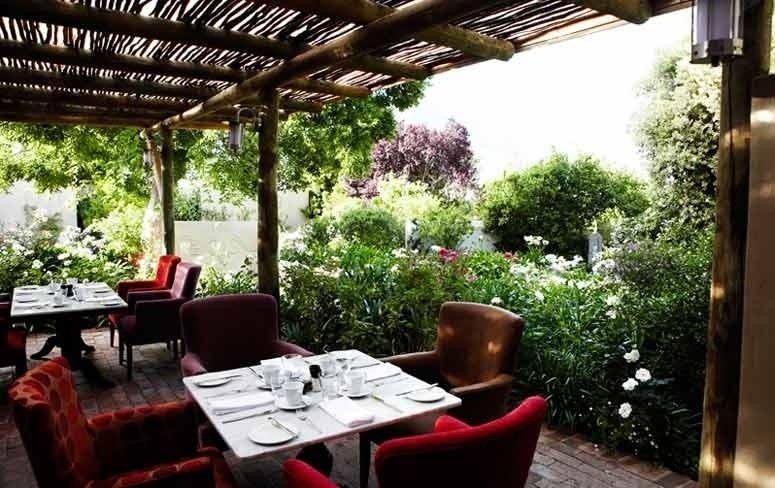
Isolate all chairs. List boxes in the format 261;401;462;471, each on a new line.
359;301;525;488
120;261;202;379
107;255;181;365
6;357;240;488
181;294;315;452
281;395;548;487
0;302;27;376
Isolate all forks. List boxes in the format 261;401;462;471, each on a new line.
295;409;322;434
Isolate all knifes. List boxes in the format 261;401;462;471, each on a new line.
271;419;299;440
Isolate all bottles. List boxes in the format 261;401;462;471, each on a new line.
310;364;321;392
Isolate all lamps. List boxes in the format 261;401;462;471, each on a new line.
691;1;743;64
229;117;243;153
143;149;151;165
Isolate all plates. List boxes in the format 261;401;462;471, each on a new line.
249;422;299;445
16;296;39;302
193;374;231;386
407;388;445;401
100;303;120;306
19;286;36;289
328;351;349;359
344;385;374;397
275;395;311;409
51;302;69;307
257;384;284;390
94;289;111;292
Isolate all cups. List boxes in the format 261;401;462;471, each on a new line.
48;279;56;292
345;371;363;394
73;277;78;287
281;378;304;406
66;278;72;284
54;295;65;303
282;354;310;378
320;378;337;397
260;359;282;385
319;357;336;376
